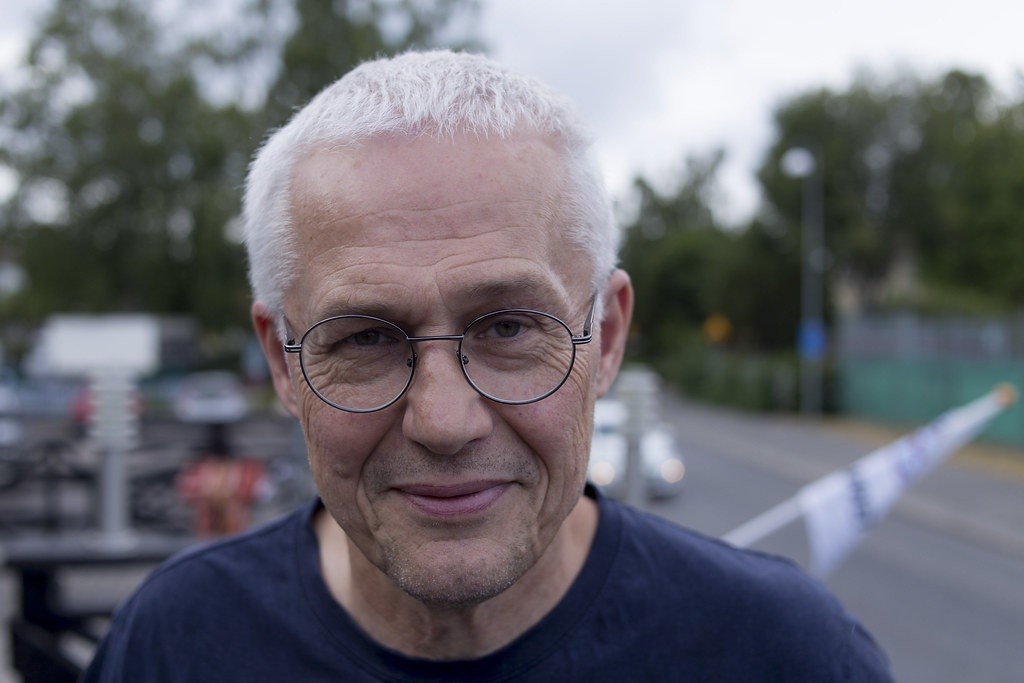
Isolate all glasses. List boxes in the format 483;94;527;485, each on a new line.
281;288;600;416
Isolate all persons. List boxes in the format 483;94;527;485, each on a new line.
80;49;896;683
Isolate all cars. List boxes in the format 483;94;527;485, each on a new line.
580;365;690;507
